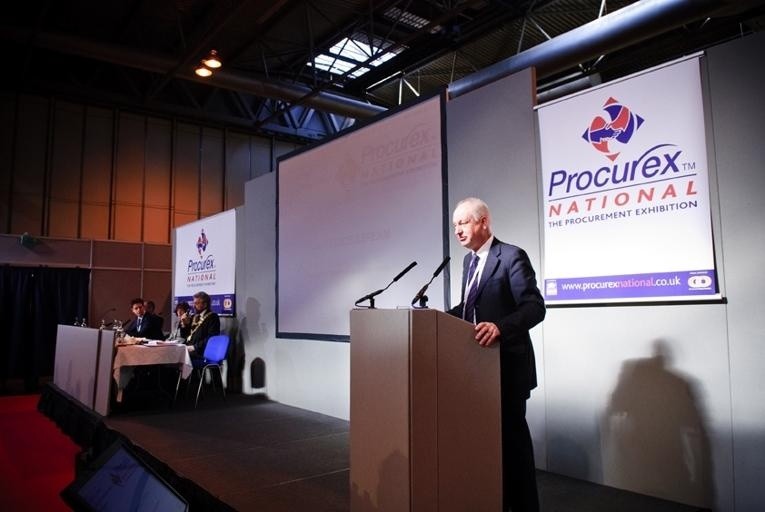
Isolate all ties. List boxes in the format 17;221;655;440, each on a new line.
461;254;480;325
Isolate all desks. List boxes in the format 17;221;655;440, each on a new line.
111;335;195;409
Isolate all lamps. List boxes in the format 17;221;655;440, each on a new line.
195;47;223;78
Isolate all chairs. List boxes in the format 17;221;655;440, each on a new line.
171;334;231;410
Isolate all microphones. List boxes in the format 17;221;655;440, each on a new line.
412;256;450;308
184;309;190;317
99;308;116;320
355;261;417;308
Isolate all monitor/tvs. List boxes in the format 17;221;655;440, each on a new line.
60;437;189;512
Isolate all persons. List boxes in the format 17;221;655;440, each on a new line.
142;300;164;331
443;197;547;510
161;301;190;344
125;297;164;341
179;292;220;405
